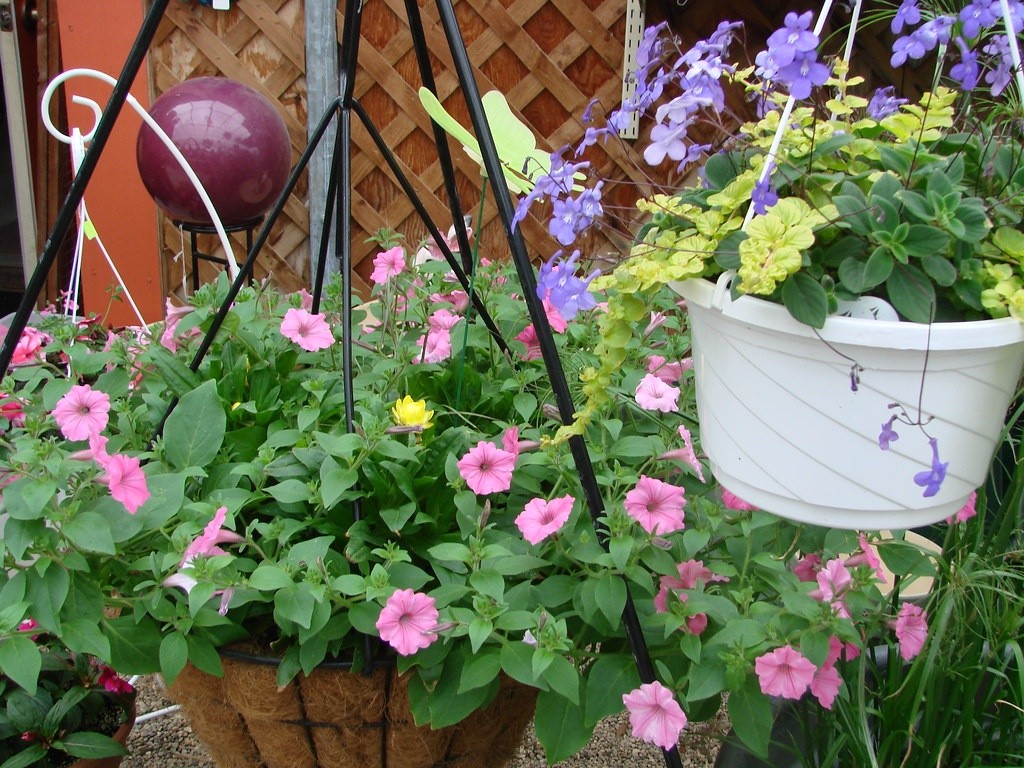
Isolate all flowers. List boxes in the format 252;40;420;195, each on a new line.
0;0;1024;768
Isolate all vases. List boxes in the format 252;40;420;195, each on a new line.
668;277;1024;532
160;647;542;768
